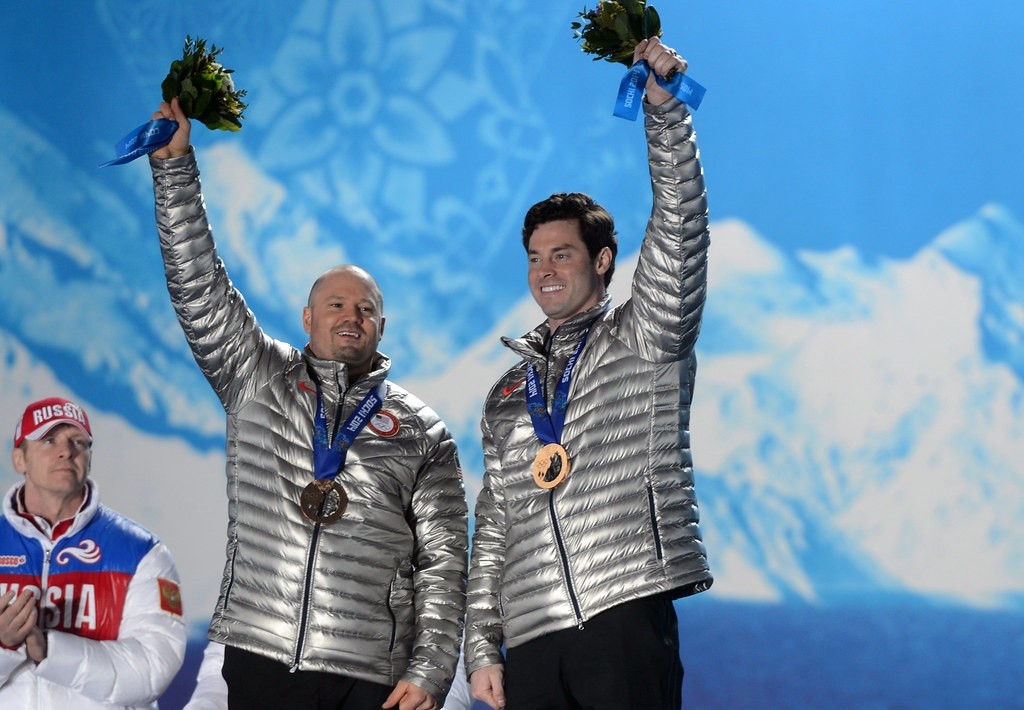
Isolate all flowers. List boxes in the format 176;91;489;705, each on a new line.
571;0;678;84
162;34;251;133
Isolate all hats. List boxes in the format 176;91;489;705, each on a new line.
14;398;93;448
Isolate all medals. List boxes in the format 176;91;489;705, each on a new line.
530;442;572;490
300;478;350;525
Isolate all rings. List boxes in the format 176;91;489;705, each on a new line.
670;50;677;56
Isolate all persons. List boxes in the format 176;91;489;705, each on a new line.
462;34;711;709
0;397;186;710
143;95;468;709
